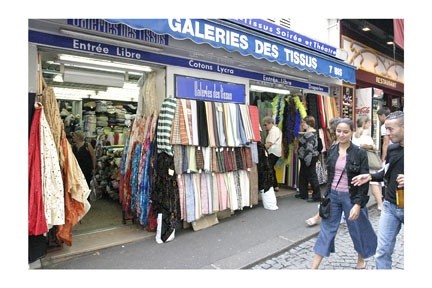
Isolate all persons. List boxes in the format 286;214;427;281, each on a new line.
306;108;393;226
72;130;96;203
295;116;323;202
262;116;282;192
351;111;404;270
306;117;378;270
151;151;181;244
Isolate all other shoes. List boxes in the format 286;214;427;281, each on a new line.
295;194;306;199
308;198;319;202
306;218;318;225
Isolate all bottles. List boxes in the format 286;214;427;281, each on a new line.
396;174;404;208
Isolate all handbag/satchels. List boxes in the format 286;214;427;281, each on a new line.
367;149;382;169
319;197;330;218
89;175;102;202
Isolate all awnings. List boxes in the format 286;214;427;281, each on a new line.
103;19;357;84
